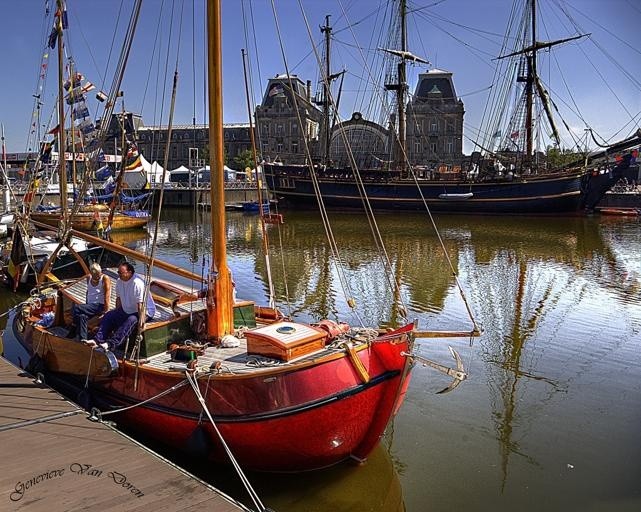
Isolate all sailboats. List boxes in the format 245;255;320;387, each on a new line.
260;0;641;210
153;196;641;494
1;0;489;474
220;439;411;512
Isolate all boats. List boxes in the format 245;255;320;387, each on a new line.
195;192;274;213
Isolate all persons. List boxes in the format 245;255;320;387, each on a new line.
66;263;111;345
85;261;155;351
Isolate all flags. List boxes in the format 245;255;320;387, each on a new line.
73;71;86;82
82;137;101;154
64;88;84;105
81;81;95;92
70;104;89;121
77;119;95;135
102;175;116;195
85;147;105;165
96;92;108;103
63;78;81;91
123;155;143;173
94;165;112;181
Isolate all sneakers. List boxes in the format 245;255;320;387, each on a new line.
99;342;111;352
80;338;97;347
